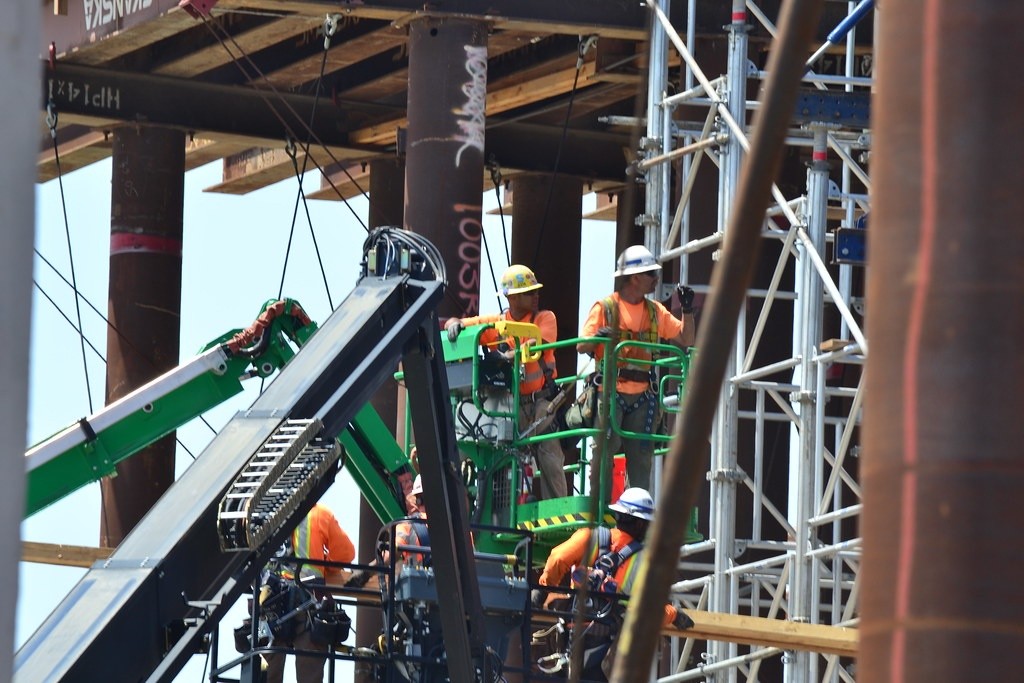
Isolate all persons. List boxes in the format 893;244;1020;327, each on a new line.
531;487;695;683
344;473;480;588
576;243;697;511
261;495;355;683
443;263;570;501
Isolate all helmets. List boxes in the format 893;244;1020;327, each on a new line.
494;264;543;296
613;244;662;278
607;487;654;521
411;474;423;496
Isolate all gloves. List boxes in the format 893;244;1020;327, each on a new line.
671;604;695;631
678;286;695;313
594;326;614;337
343;572;369;586
531;588;548;608
447;322;466;342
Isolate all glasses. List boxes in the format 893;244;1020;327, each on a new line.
636;269;659;278
523;290;538;295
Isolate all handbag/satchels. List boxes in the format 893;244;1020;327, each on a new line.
565;385;598;429
